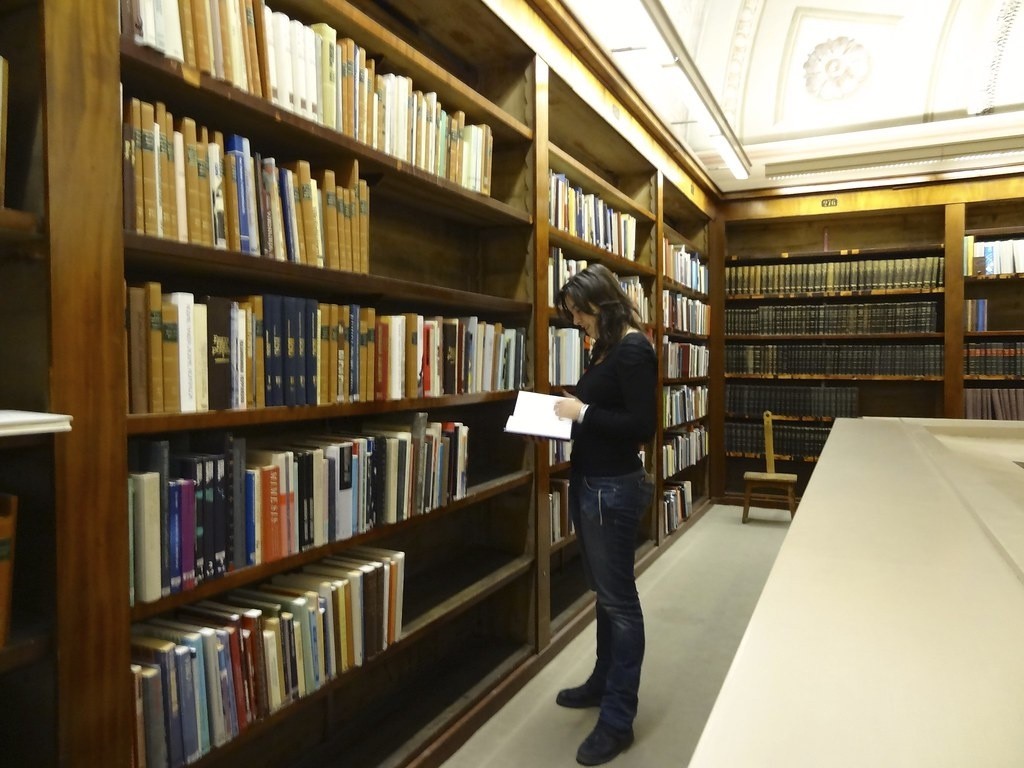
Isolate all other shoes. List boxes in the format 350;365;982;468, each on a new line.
555;683;601;707
576;719;634;767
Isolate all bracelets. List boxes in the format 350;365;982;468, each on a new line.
578;403;589;424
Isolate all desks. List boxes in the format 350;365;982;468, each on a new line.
688;417;1024;768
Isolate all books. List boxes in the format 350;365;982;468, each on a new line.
117;0;713;768
725;236;1024;462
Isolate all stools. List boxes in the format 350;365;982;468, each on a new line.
742;471;798;524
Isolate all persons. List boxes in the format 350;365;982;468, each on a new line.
555;262;658;765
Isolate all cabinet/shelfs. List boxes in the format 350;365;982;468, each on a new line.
0;0;1024;768
